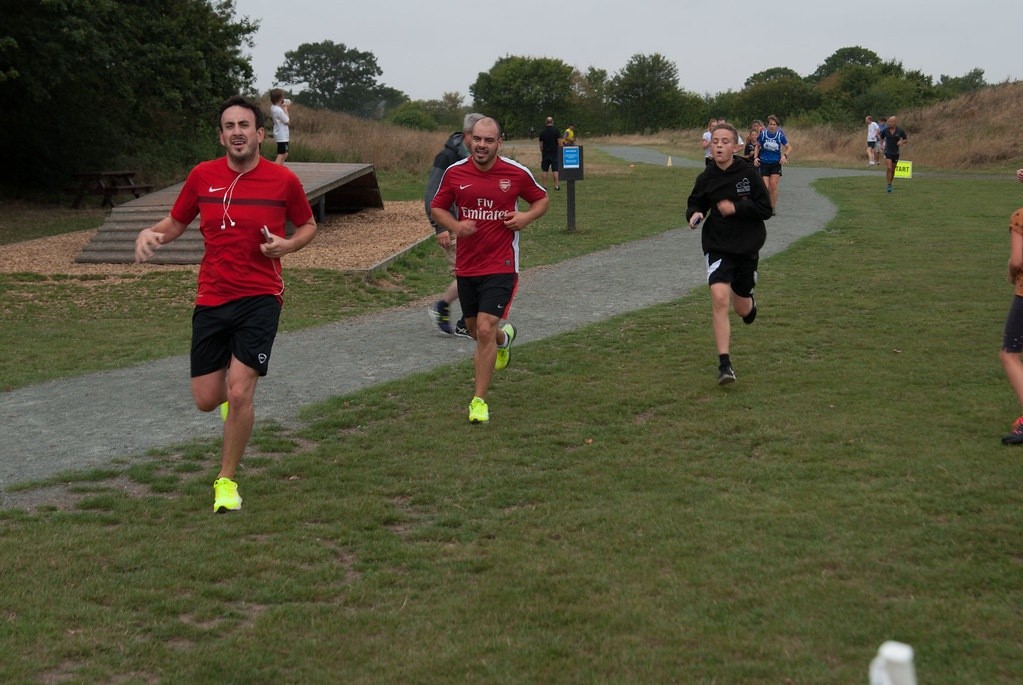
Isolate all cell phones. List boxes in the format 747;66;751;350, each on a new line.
694;216;700;226
264;225;273;244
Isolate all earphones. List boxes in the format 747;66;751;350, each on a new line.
230;218;235;227
221;221;225;229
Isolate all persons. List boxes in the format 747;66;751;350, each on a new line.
1001;207;1023;444
685;124;773;385
134;95;318;512
270;90;290;164
866;116;889;166
431;117;549;424
562;124;575;147
745;116;792;215
539;117;564;190
424;113;486;338
880;116;908;192
702;118;745;167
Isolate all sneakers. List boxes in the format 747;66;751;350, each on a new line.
214;477;243;513
717;363;736;386
468;396;489;424
219;401;228;422
494;324;516;369
743;293;758;325
1001;416;1023;445
428;299;454;336
454;320;475;339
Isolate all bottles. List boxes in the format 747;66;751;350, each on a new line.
280;99;291;106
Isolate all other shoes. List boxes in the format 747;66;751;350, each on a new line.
867;161;875;165
887;184;892;192
772;207;776;216
